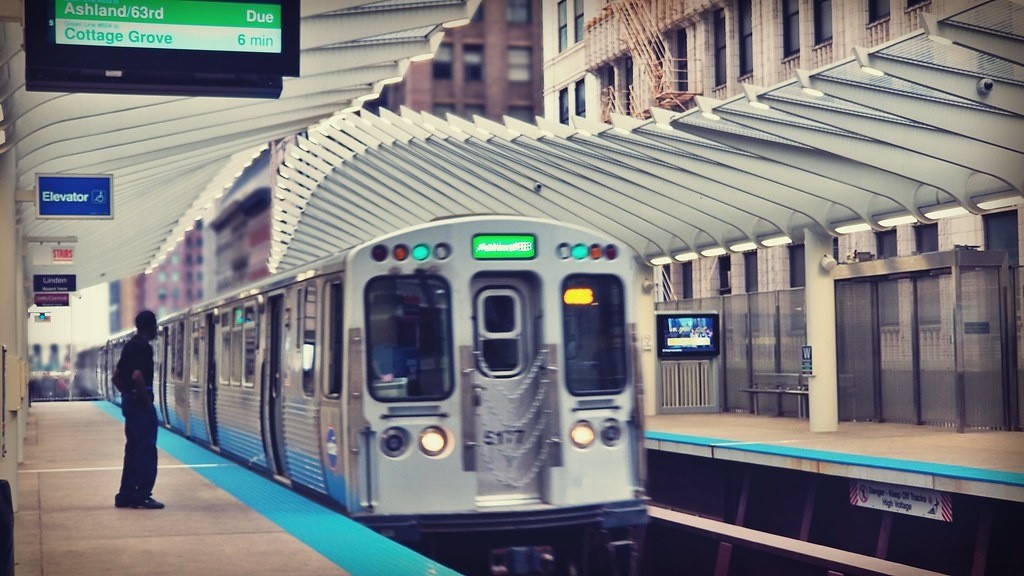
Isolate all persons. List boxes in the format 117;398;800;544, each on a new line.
112;310;164;509
371;320;400;382
668;327;711;348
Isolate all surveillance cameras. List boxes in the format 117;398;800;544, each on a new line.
976;78;994;93
534;182;542;191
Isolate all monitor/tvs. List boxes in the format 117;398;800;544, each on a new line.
656;314;720;357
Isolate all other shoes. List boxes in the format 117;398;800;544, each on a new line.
115;500;129;507
130;496;164;508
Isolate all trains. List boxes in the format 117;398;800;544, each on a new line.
76;213;653;576
